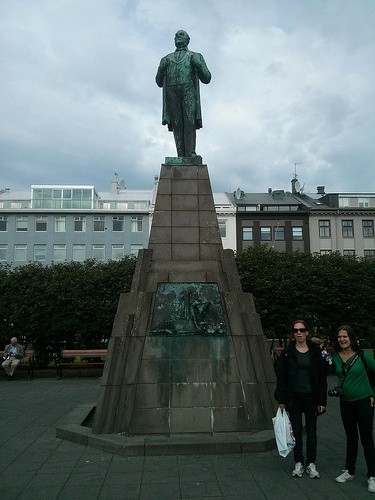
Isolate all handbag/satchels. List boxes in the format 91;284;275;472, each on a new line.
272;407;296;458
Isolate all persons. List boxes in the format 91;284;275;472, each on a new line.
274;321;327;478
311;337;329;360
332;325;375;492
156;30;212;156
2;337;24;380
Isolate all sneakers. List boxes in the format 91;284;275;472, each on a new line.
335;470;355;483
292;462;304;478
367;477;375;492
306;463;320;479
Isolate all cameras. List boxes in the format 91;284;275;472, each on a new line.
328;386;345;398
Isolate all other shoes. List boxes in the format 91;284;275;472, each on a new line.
8;375;12;381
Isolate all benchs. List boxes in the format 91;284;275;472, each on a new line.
0;350;35;379
57;350;107;380
274;347;334;369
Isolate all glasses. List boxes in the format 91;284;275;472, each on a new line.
293;328;307;333
